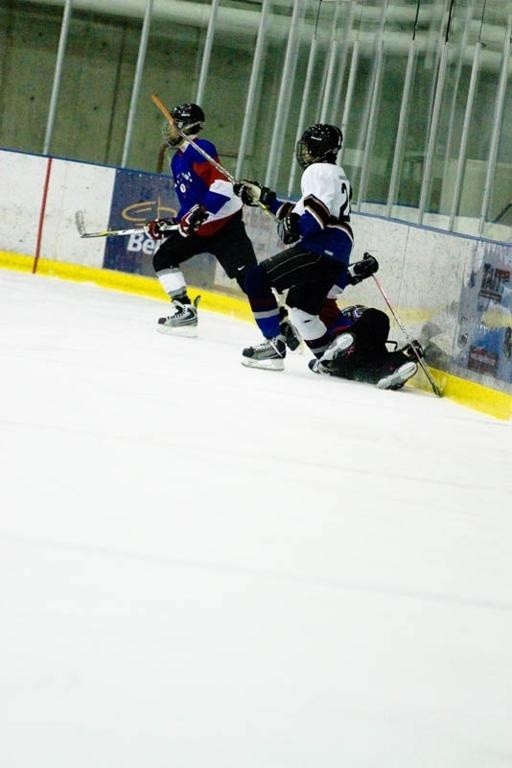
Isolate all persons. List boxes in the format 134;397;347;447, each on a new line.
423;265;506;382
309;306;422;391
234;123;355;372
144;103;284;340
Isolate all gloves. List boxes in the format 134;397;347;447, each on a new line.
177;203;209;239
232;178;276;207
276;216;301;245
144;217;176;240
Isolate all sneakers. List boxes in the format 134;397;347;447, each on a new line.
240;337;286;372
154;294;202;339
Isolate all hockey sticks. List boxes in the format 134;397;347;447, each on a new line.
371;271;447;397
75;211;179;239
151;92;281;222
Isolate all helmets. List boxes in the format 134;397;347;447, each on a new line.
295;124;343;170
162;103;206;148
